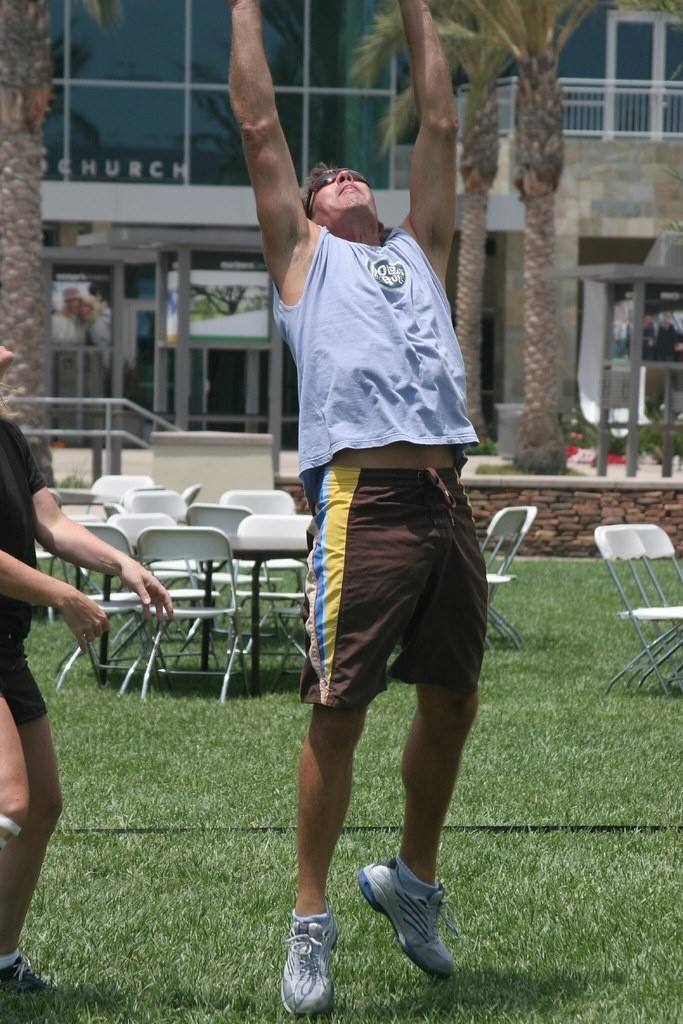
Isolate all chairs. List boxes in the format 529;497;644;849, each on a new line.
35;475;314;705
595;524;683;698
481;505;538;654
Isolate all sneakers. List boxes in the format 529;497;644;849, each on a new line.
0;955;52;995
281;897;337;1016
357;856;459;978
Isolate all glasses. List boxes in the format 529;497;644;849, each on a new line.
306;169;370;218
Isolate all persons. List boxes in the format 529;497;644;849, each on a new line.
0;346;176;995
57;287;98;325
228;0;488;1016
627;314;655;360
656;314;677;362
